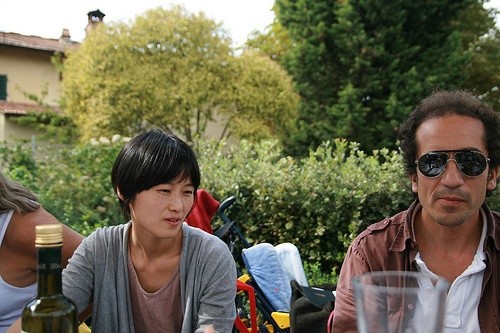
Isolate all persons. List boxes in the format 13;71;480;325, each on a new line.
331;92;500;333
6;132;236;332
0;175;85;333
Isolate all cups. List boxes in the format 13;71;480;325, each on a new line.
351;271;447;333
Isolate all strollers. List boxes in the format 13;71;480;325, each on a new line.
182;190;336;333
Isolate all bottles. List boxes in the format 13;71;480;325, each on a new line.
21;224;78;333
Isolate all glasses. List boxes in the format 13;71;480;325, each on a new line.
415;150;490;178
